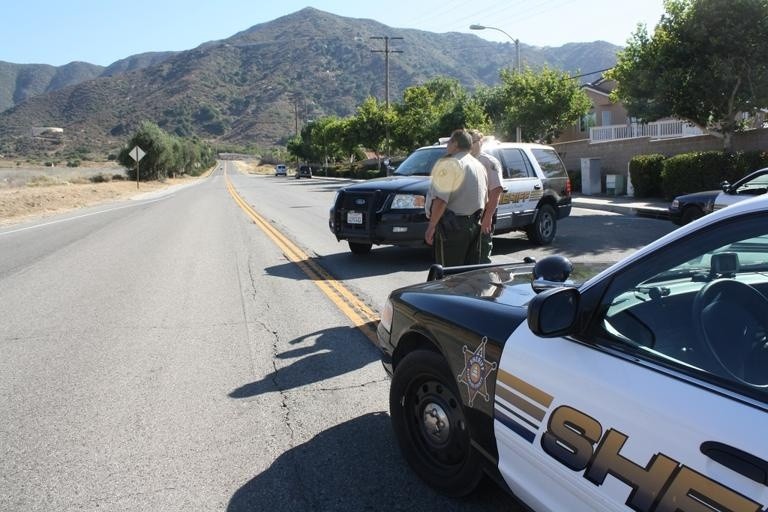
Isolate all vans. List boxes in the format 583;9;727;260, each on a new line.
274;164;287;177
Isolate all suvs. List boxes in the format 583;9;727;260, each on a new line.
295;165;312;179
329;135;573;260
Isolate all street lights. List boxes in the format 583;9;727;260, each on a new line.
469;25;521;142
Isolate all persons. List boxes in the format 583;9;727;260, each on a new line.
423;128;489;280
424;151;451;266
466;129;503;265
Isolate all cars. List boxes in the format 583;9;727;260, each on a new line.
376;192;768;511
669;167;768;227
